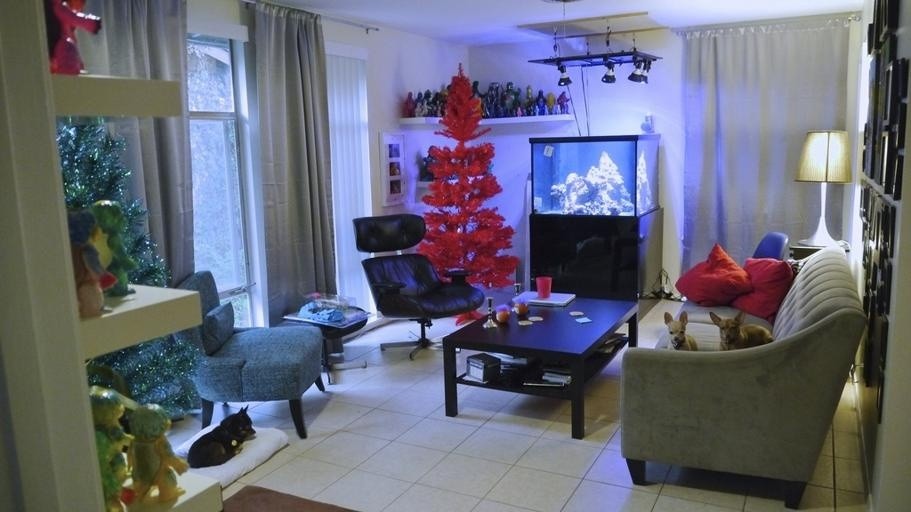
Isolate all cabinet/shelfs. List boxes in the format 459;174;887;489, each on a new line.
528;134;664;324
1;2;224;512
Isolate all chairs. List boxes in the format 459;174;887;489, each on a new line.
171;270;326;439
353;214;484;361
751;232;789;261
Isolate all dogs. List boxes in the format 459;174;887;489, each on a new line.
187;404;256;469
709;309;775;351
663;310;699;351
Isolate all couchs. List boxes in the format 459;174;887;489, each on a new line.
620;246;868;509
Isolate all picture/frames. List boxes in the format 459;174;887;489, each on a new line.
379;130;407;207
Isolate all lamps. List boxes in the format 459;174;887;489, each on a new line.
528;18;664;87
793;130;852;248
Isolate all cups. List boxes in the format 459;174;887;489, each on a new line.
536;276;553;298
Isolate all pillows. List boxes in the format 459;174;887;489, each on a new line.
203;301;234;356
675;242;753;309
732;257;794;326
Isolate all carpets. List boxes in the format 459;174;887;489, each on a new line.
223;485;359;512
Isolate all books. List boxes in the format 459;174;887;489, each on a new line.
523;369;572;388
514;291;576;307
596;334;626;354
